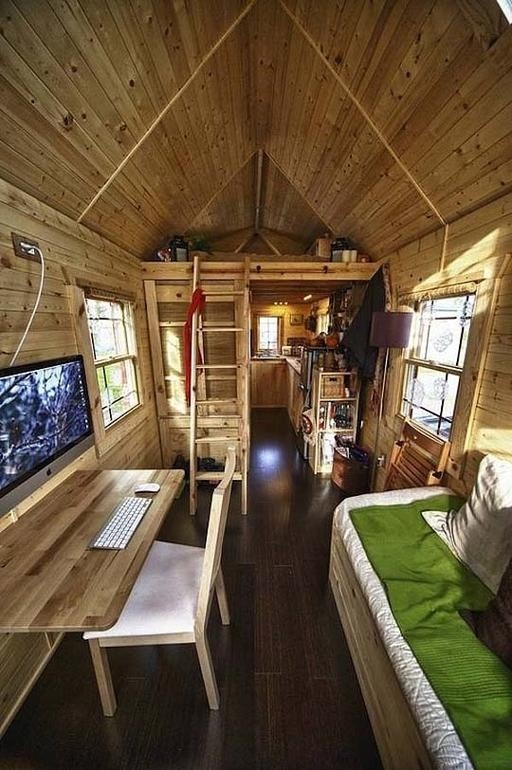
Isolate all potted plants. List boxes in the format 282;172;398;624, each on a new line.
188;239;214;262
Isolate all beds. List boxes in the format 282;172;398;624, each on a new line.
328;487;512;770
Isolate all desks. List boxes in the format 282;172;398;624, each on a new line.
0;469;185;633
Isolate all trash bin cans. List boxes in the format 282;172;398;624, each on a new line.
330;446;370;498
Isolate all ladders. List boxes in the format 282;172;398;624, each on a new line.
188;255;251;515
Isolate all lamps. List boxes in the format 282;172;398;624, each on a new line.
369;312;413;493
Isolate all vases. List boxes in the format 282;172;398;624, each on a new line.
176;248;187;262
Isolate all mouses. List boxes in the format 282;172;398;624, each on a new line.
135;483;160;497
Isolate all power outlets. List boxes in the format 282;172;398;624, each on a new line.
12;232;42;262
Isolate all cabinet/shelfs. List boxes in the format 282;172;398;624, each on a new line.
251;361;287;408
308;364;362;475
287;363;303;437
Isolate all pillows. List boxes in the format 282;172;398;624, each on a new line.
458;557;512;672
422;453;512;596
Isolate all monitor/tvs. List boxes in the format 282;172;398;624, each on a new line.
0;354;94;516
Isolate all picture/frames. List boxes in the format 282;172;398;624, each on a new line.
290;314;303;325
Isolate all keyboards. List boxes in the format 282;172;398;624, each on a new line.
89;496;153;550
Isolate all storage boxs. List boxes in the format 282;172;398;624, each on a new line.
316;239;332;258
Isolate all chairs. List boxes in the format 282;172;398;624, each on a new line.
83;448;236;717
381;416;451;492
